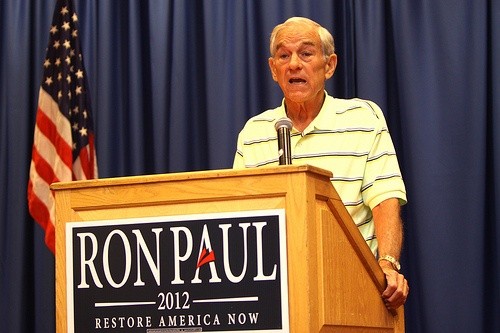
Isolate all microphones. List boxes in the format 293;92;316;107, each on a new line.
275;116;293;165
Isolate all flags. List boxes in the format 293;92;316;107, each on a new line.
28;0;99;257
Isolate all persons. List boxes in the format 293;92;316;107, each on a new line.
232;15;410;311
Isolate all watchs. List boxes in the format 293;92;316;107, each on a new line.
378;254;402;274
